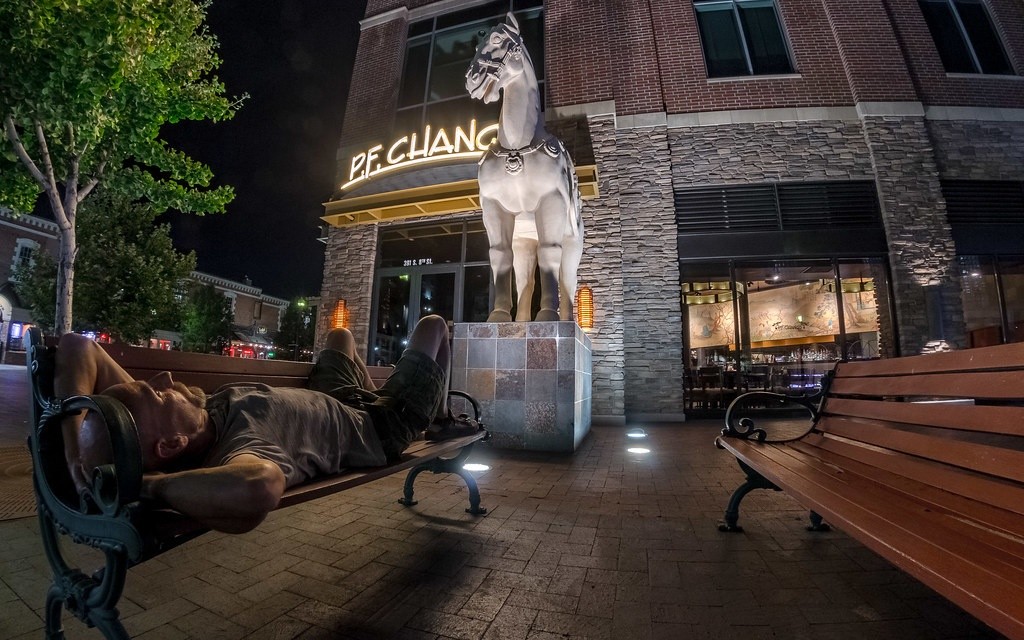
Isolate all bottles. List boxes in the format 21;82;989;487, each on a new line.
714;350;718;361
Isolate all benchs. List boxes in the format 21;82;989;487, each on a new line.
25;326;493;640
714;342;1024;640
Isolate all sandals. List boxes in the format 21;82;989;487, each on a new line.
425;408;479;440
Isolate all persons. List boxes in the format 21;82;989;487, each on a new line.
52;314;493;535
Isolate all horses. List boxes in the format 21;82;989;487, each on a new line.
464;9;584;323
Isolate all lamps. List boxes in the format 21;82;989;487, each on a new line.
578;286;594;328
681;281;744;305
815;278;876;294
337;299;347;328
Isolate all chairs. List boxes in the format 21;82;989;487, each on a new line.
684;363;795;411
